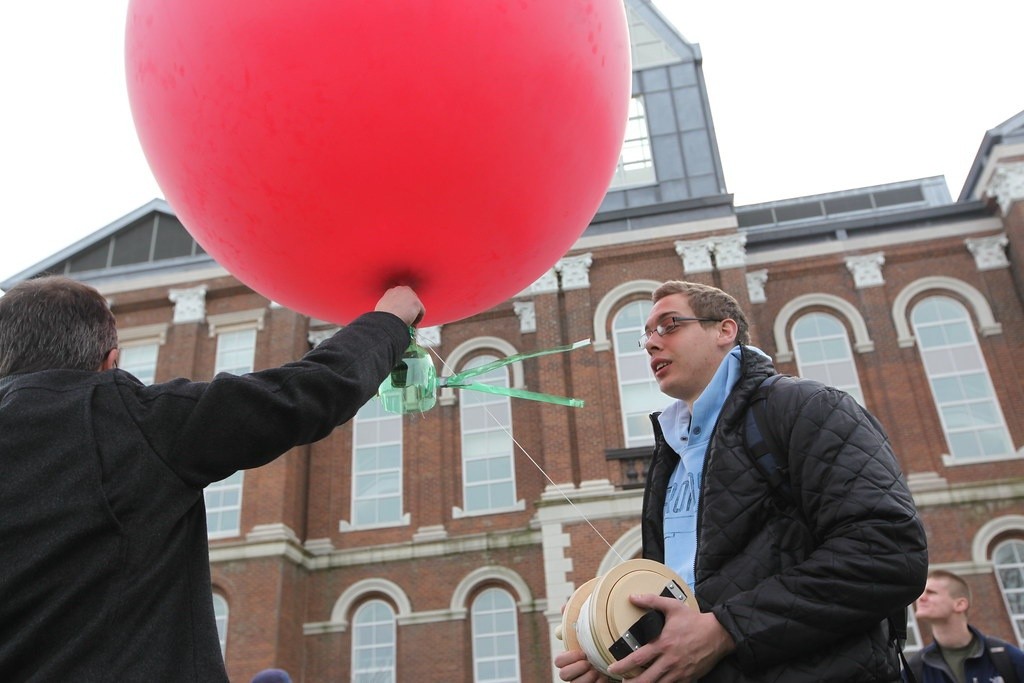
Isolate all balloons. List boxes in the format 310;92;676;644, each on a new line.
124;0;633;329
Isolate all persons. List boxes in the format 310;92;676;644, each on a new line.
554;279;927;683
0;277;425;683
898;570;1024;683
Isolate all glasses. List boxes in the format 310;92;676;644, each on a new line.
638;316;722;349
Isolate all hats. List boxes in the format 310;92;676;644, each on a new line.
252;669;292;683
